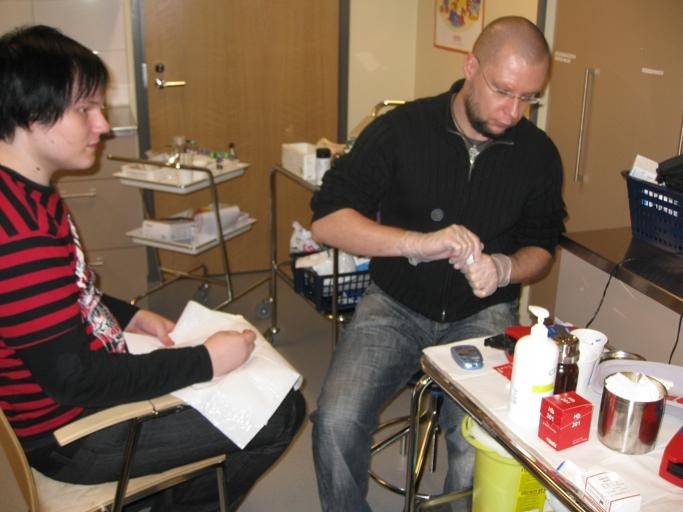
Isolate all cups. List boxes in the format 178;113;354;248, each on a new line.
597;373;667;456
569;329;608;395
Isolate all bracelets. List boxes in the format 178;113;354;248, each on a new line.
491;256;501;283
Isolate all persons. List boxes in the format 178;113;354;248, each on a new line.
0;20;308;512
308;13;568;512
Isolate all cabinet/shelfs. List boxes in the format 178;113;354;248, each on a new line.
262;165;338;352
403;333;681;511
51;134;150;302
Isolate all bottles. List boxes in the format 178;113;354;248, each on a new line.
316;148;331;186
552;331;579;394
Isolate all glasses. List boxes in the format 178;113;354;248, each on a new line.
474;55;543;106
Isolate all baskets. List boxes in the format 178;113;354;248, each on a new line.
289;250;370;313
620;168;683;259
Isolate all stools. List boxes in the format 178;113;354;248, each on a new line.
367;370;446;501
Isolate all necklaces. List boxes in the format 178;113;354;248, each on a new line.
451;93;492;165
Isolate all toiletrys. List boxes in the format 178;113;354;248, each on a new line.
505;302;559;419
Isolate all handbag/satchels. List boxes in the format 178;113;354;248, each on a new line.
655;154;683;192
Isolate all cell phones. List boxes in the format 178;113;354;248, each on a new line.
451;344;484;370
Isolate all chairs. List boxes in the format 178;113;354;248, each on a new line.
0;391;227;512
